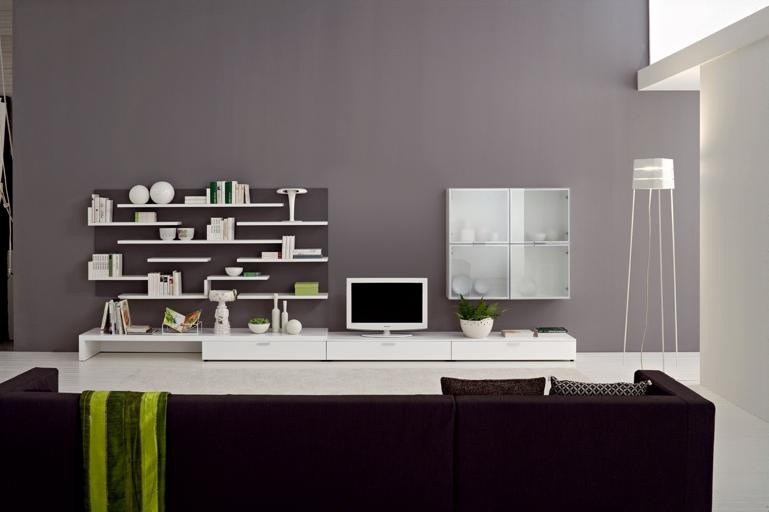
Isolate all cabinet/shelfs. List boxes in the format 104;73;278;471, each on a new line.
80;329;327;363
326;331;579;365
80;185;331;321
444;186;571;301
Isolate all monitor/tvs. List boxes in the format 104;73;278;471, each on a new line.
346;278;428;337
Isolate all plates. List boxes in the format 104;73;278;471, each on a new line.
474;279;489;293
452;272;473;294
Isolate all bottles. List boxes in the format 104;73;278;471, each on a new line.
281;300;288;334
213;297;231;335
461;217;475;242
491;232;499;241
271;293;280;333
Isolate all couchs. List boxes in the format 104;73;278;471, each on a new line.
2;366;718;511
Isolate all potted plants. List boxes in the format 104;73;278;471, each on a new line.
452;289;503;339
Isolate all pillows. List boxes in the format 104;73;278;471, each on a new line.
550;371;657;397
441;371;547;395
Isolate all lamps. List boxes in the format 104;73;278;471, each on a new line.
625;156;684;359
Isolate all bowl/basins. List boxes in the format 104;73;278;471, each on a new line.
225;265;243;276
248;322;269;334
177;227;195;240
530;233;546;241
160;227;176;241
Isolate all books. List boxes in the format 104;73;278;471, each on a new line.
90;193;114;222
148;270;182;296
92;253;124;278
262;234;324;259
206;217;235;241
185;177;253;204
502;322;567;339
100;300;149;336
162;307;203;332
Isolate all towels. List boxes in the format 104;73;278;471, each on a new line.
77;388;172;510
